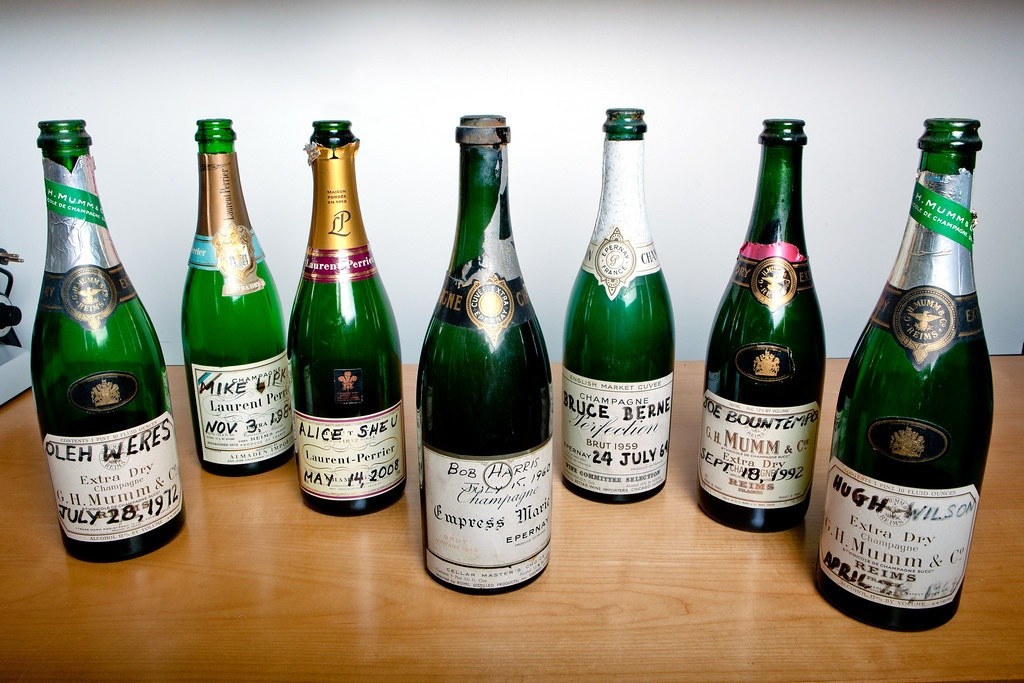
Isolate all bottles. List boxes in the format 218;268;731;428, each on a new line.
409;121;553;595
811;117;993;633
180;117;294;477
697;116;827;530
26;119;189;564
284;117;406;518
561;108;675;505
